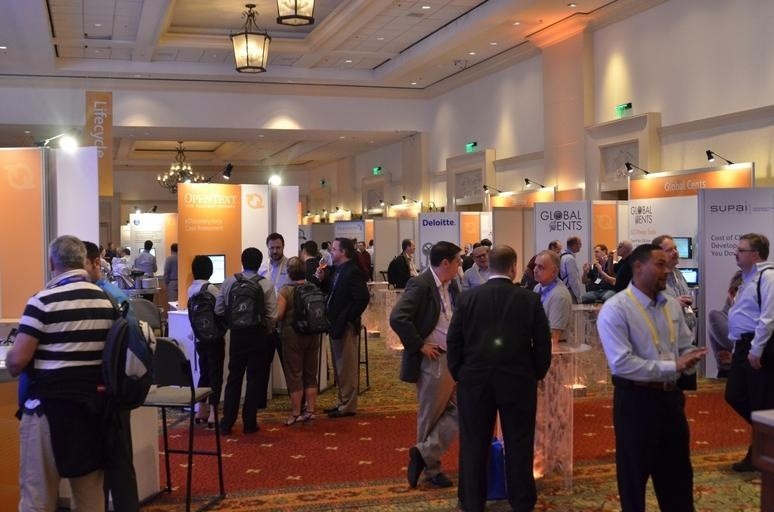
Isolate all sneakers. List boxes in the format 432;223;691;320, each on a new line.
734;446;754;471
324;407;355;417
220;418;257;434
407;447;453;489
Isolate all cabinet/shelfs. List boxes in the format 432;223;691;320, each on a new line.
272;335;328;396
751;410;774;512
165;308;225;414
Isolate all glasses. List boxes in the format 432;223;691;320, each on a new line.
736;248;756;253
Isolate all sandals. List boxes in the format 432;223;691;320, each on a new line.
284;409;315;425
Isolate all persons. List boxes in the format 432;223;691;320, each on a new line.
99;240;179;311
387;239;492;489
3;234;144;511
215;247;278;435
446;245;553;512
597;244;709;511
709;270;744;379
522;235;696;345
261;232;375;425
187;254;226;424
532;250;573;471
725;233;774;473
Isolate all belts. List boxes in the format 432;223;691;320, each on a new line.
634;380;677;391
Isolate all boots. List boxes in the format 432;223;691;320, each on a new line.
196;402;217;427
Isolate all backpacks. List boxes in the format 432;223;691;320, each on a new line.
188;283;228;343
283;279;332;335
226;272;266;330
387;256;403;287
100;288;156;415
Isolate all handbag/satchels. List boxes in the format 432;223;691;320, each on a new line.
486;438;507;500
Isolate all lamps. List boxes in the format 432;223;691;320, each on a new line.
483;184;502;194
624;161;650;174
307;196;417;216
230;3;271;73
222;163;233;180
706;149;735;165
524;178;546;188
276;0;316;26
157;139;205;193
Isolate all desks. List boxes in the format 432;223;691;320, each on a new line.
533;304;612;496
360;281;407;351
121;287;163;306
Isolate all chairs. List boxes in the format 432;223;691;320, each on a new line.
130;335;226;512
123;298;169;338
317;325;371;396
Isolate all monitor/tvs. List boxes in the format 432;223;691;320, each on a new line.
678;268;698;286
672;236;692;259
138;247;156;256
207;254;227;284
